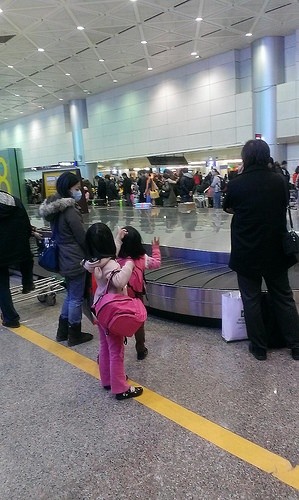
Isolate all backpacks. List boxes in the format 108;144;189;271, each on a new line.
90;269;147;338
216;176;225;190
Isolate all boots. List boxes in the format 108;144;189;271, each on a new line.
56;314;93;347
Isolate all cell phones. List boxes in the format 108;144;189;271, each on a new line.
255;134;261;139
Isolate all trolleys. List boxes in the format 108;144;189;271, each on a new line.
0;282;65;320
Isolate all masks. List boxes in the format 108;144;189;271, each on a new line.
70;190;83;201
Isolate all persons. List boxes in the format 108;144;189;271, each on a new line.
80;223;162;400
24;160;299;209
222;139;299;362
0;190;35;328
39;171;93;346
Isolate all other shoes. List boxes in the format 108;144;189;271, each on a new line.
104;375;143;400
137;347;148;359
22;284;35;294
249;343;267;360
290;348;299;359
2;320;20;328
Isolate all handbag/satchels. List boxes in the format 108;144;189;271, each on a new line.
38;244;59;273
221;290;248;341
149;189;159;198
159;189;171;198
287;232;299;270
